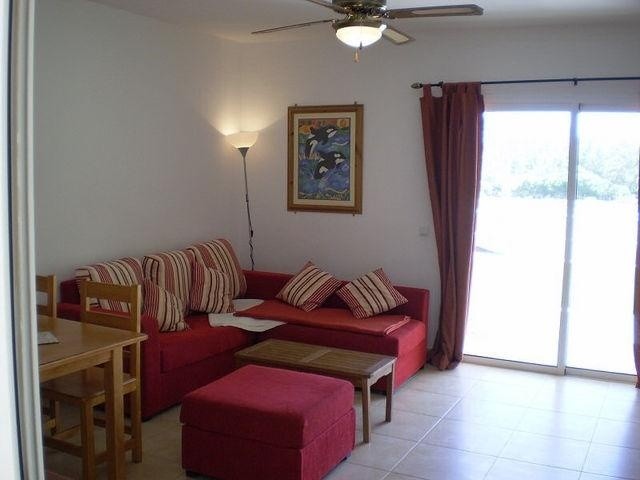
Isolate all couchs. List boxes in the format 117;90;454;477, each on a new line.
61;267;430;420
178;365;357;480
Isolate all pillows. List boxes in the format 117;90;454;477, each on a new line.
187;238;248;297
278;257;344;310
339;269;407;318
142;251;194;312
74;256;145;313
139;279;191;333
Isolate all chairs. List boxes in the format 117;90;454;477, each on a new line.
33;275;59;317
40;278;142;480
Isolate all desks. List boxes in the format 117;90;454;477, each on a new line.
30;313;149;480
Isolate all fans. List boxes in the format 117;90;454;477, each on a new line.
249;0;485;45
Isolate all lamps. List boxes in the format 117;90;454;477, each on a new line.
226;133;259;270
332;20;387;63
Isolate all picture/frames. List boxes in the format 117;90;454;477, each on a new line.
287;104;365;217
